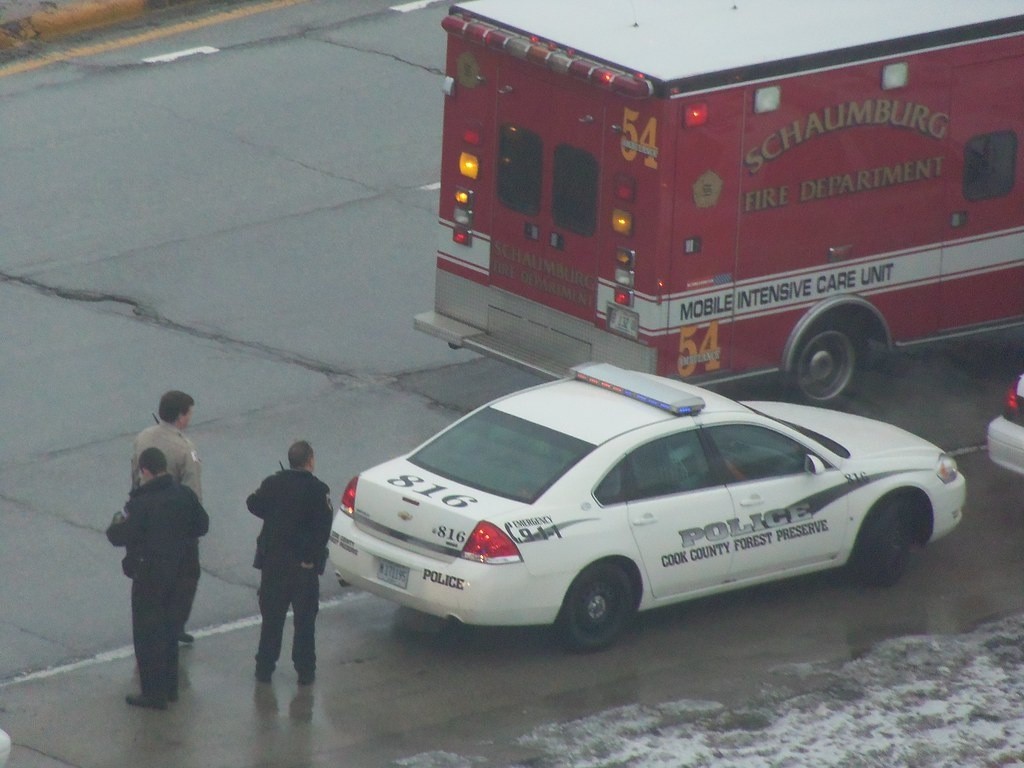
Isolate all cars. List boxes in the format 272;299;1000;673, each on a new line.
987;373;1024;474
328;360;966;653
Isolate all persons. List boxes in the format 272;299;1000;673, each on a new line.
245;439;333;685
131;389;202;643
106;447;209;708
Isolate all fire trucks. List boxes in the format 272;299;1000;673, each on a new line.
412;0;1024;406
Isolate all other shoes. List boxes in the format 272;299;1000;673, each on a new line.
298;674;314;684
126;694;167;710
254;670;271;683
178;633;194;642
